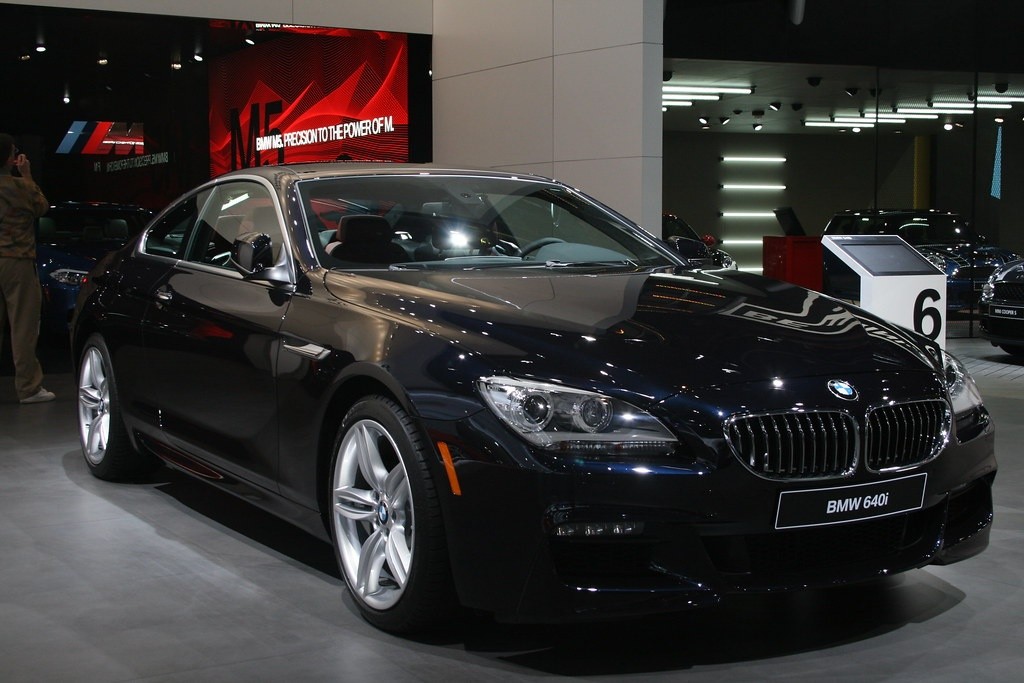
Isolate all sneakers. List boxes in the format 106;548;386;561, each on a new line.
20;385;56;403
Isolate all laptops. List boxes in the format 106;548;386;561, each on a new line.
773;207;807;236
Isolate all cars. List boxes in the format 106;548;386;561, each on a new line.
35;201;156;371
978;258;1024;354
661;213;738;272
76;162;998;634
822;208;1020;321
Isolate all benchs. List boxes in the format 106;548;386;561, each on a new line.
55;224;103;242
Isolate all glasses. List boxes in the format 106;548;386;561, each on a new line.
10;147;19;156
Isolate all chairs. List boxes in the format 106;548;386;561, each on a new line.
105;219;130;241
214;214;244;261
325;215;414;263
415;222;502;259
37;218;57;245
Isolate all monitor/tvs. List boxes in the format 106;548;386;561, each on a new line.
843;243;934;272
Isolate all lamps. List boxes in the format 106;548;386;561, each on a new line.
769;103;782;110
663;85;756;95
720;116;730;125
845;88;858;97
719;211;776;217
799;120;875;128
891;104;974;115
927;98;1012;109
720;156;788;163
753;123;762;131
718;239;763;244
699;116;710;124
721;184;787;190
829;114;909;124
859;110;940;119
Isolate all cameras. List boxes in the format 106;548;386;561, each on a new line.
14;157;22;165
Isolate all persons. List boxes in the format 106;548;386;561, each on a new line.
0;134;55;404
320;155;381;214
